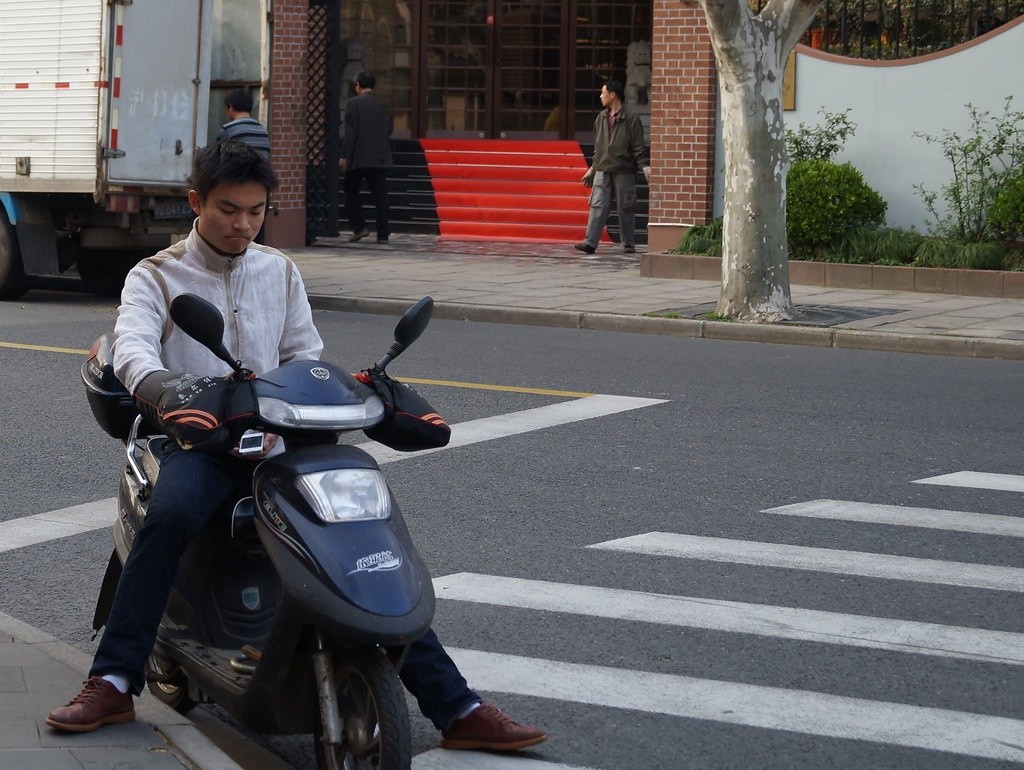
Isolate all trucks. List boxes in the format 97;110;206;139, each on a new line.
0;1;276;297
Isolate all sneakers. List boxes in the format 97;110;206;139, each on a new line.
45;677;138;733
438;704;548;751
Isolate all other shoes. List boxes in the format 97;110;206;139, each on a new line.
625;245;634;256
349;228;369;242
376;235;389;244
575;242;596;256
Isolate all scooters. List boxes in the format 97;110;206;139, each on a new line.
80;294;439;770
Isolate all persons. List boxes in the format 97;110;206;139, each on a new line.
44;141;549;750
425;45;483;133
573;81;650;254
340;71;394;244
213;92;270;244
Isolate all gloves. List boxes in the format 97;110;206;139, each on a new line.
643;166;653;182
582;166;595;190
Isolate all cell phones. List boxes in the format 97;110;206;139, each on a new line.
239;431;265;455
